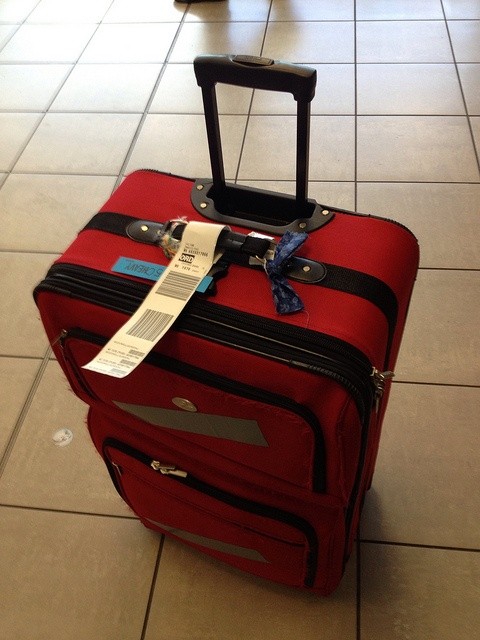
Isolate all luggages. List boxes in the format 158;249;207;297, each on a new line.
32;55;417;599
